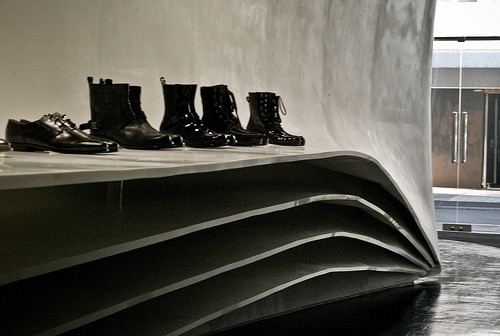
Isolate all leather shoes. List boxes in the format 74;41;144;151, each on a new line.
6;115;110;156
18;111;120;154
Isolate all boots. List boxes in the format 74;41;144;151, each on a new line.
86;76;169;149
199;84;269;147
244;89;305;148
105;79;186;149
159;76;223;149
177;83;237;150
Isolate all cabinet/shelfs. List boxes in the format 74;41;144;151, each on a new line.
0;148;442;336
